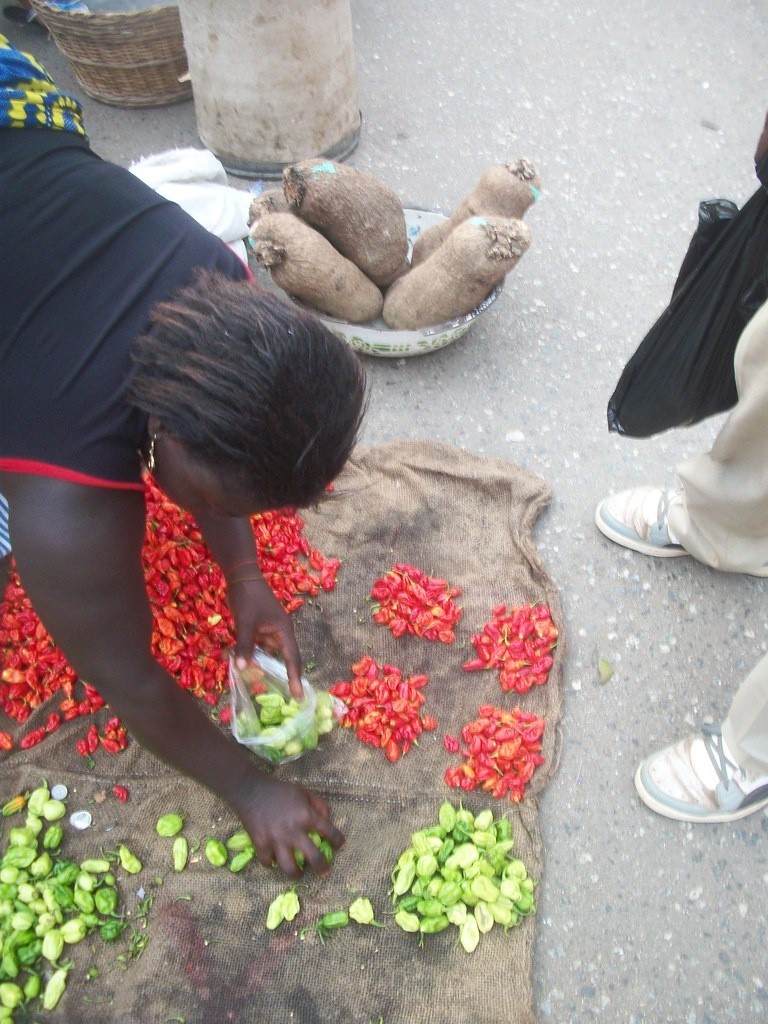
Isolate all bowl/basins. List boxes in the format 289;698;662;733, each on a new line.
282;209;506;359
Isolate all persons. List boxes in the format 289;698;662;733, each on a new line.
594;300;768;823
0;32;365;882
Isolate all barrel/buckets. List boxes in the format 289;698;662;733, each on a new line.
178;0;363;182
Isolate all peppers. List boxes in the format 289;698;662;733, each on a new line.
0;473;559;1024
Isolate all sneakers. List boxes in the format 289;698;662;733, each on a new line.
594;484;691;558
633;727;768;823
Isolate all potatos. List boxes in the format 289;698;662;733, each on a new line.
247;156;537;331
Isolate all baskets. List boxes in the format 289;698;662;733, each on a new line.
26;0;195;109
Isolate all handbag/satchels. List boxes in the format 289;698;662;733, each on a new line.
607;152;768;440
